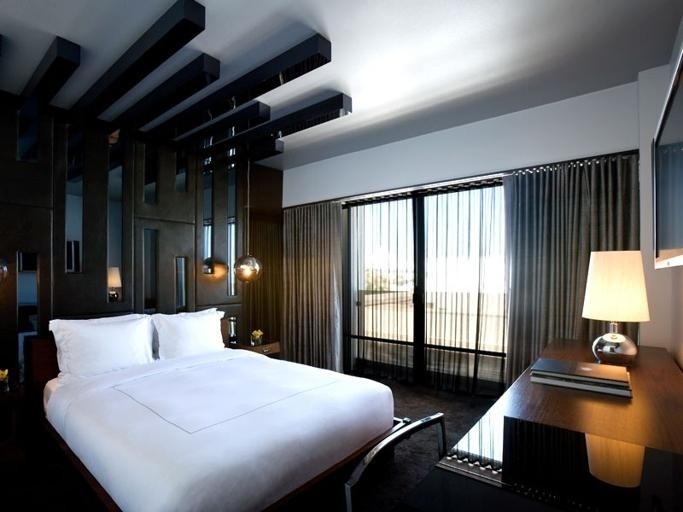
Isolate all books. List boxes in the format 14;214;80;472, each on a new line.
531;355;630;389
530;369;633;400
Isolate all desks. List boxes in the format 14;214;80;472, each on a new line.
433;334;682;511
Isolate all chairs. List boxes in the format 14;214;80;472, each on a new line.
343;411;447;512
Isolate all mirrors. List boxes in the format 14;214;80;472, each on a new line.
106;165;124;304
64;170;84;275
226;184;237;296
201;170;215;274
174;255;187;312
143;228;159;311
14;249;40;383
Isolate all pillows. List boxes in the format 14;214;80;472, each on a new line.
48;307;227;385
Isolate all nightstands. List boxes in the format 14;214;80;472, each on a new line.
229;338;281;354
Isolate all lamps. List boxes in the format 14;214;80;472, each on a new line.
201;156;228;283
108;267;122;300
234;162;263;285
581;249;651;365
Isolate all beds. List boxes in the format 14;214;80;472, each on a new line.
43;346;411;512
